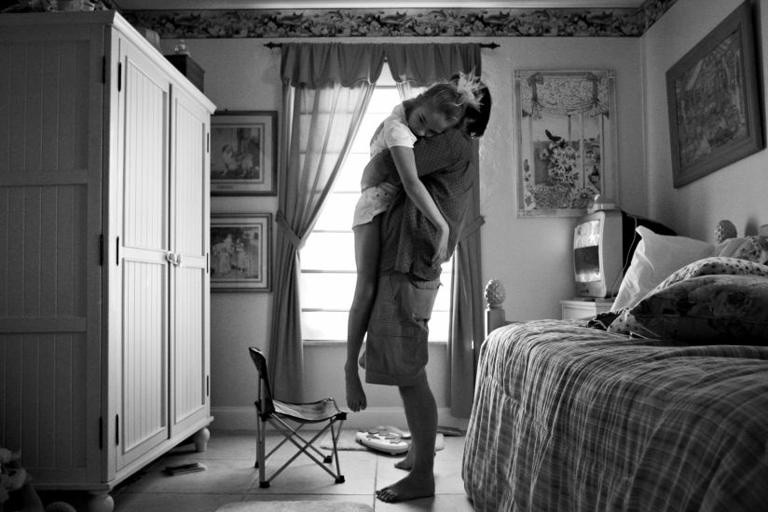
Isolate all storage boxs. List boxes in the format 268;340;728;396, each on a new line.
163;54;205;92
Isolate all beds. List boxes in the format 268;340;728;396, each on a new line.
484;279;767;511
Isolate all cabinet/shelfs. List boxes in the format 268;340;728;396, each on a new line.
1;11;217;511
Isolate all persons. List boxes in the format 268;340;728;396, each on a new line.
218;238;245;277
343;71;486;412
376;75;494;503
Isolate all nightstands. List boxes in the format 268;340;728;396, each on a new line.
558;300;612;319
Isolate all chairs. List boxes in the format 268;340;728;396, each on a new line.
249;347;347;489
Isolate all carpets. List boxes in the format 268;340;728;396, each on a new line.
217;500;376;512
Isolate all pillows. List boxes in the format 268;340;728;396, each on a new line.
606;225;768;340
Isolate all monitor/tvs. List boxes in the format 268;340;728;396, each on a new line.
572;209;676;303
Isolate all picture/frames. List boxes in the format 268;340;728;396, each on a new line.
210;211;274;294
211;110;278;196
514;68;621;219
666;0;768;189
